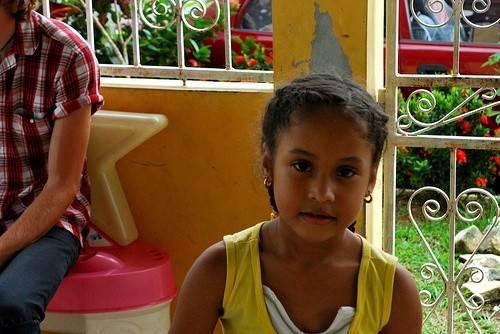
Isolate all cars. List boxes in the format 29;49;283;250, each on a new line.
136;0;500;136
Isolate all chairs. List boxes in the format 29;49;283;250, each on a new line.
38;109;182;334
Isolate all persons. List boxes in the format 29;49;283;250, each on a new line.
0;0;104;334
167;74;423;334
